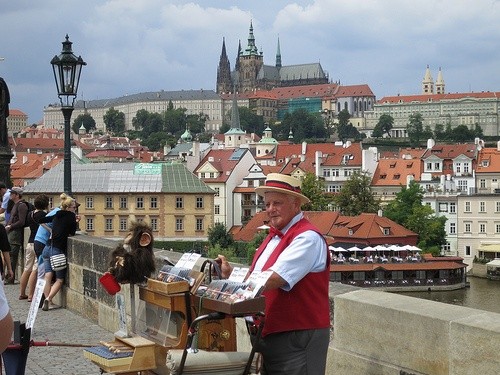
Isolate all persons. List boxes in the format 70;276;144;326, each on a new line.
4;187;28;284
214;173;330;375
19;195;51;302
34;197;80;308
36;193;77;311
0;183;12;225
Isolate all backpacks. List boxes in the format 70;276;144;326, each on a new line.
25;201;35;211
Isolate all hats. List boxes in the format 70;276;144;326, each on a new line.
254;172;310;201
9;187;23;195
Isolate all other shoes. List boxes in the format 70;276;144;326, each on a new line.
19;293;60;310
3;277;15;285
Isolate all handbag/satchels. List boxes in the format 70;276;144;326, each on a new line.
50;254;67;270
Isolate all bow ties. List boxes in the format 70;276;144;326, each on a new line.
268;225;284;240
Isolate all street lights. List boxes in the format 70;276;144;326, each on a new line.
50;33;87;196
